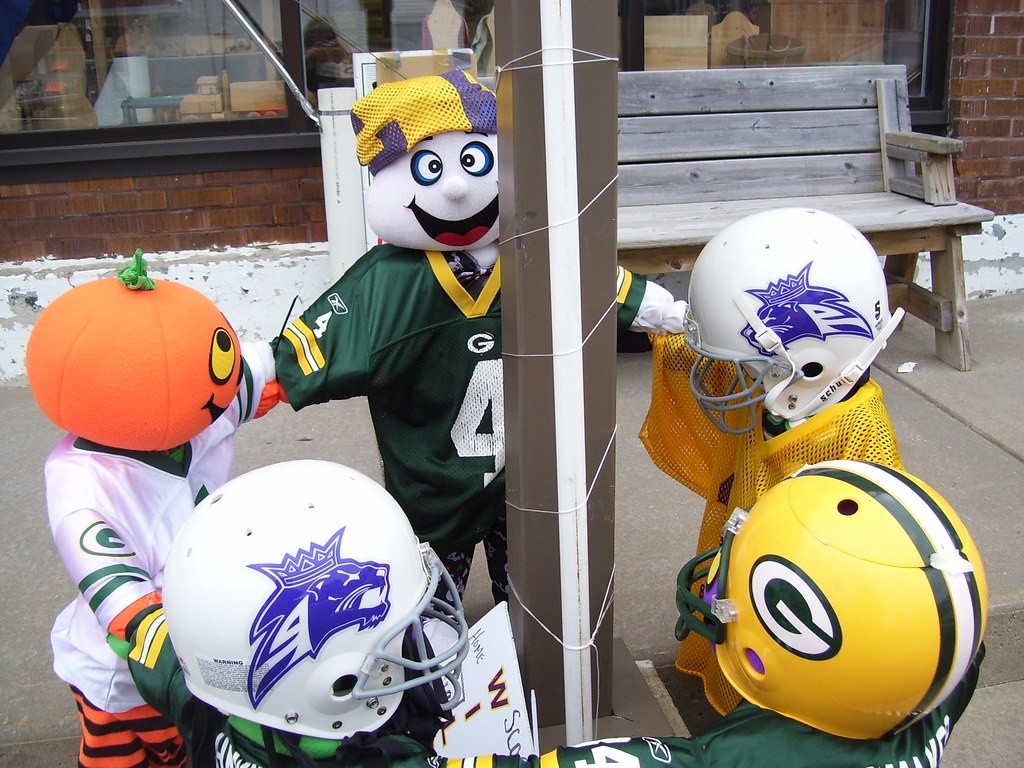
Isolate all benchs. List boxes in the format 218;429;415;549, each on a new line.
477;62;997;371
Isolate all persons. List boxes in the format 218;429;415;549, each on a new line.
256;68;691;624
125;459;529;767
529;459;988;768
637;208;907;717
24;246;276;768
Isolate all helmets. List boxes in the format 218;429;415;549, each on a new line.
674;459;989;741
161;460;470;739
682;207;906;436
